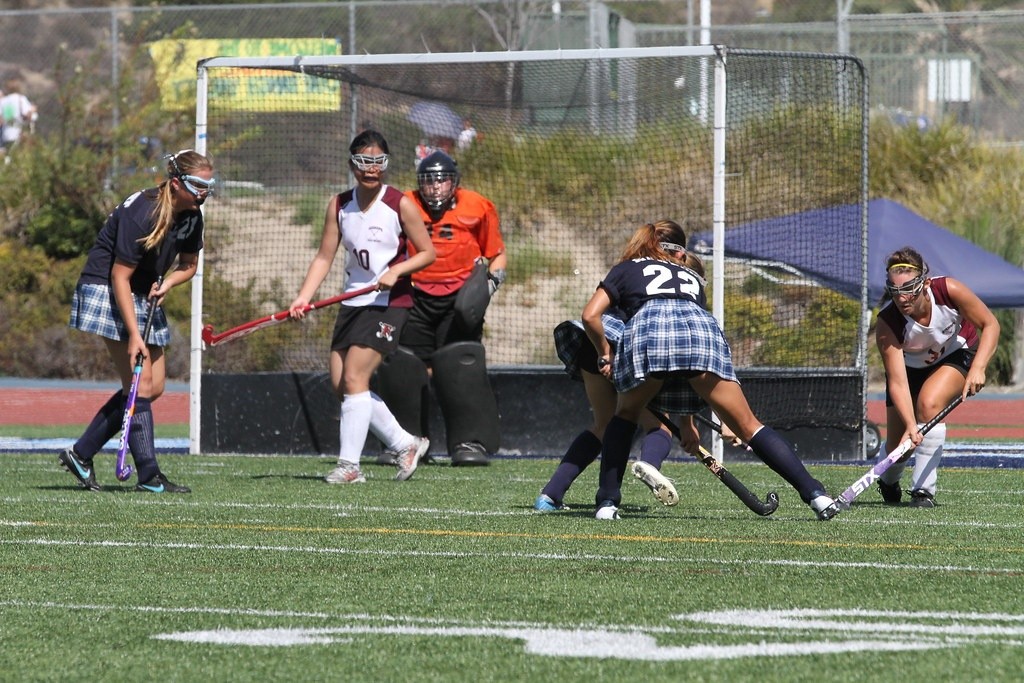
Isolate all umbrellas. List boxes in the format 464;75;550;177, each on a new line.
408;101;464;140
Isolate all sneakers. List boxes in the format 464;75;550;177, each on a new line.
376;448;398;464
394;436;430;481
877;477;901;505
906;488;941;507
631;461;679;506
534;494;571;512
595;499;622;520
451;439;488;467
324;459;365;484
59;445;101;493
135;473;191;493
809;490;840;520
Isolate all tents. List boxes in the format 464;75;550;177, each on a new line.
688;199;1024;368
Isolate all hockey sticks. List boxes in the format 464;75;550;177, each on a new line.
694;412;758;454
820;387;977;519
115;273;163;482
600;359;779;516
200;276;406;346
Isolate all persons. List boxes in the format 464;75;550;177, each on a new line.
372;152;507;469
535;253;706;512
0;78;40;164
290;129;436;483
58;149;215;494
458;115;477;149
873;246;1001;509
582;222;839;522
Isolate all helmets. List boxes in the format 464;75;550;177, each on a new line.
418;151;458;218
